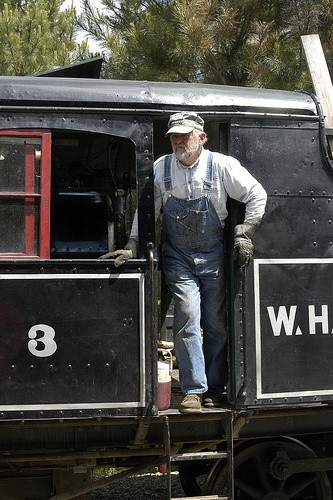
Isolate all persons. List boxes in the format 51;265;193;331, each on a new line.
98;111;267;413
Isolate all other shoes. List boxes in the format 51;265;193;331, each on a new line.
204;390;225;406
179;394;203;414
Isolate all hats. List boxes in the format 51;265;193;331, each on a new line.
166;111;204;134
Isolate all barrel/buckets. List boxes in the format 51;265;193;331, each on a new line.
157;348;173;410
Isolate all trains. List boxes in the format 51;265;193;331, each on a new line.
0;58;333;499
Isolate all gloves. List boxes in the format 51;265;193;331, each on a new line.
233;224;253;270
96;238;138;266
